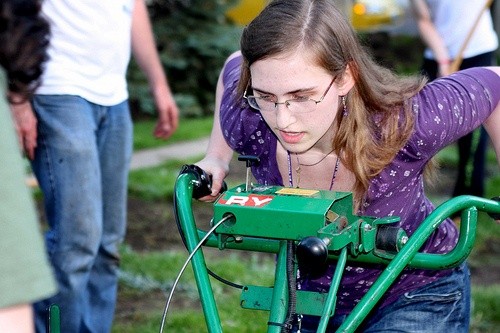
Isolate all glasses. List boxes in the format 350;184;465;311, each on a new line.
243;64;338;112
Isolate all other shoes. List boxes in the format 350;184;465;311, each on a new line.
449;164;483;198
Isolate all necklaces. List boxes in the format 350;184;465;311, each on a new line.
295;145;341;189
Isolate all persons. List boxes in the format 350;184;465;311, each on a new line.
28;0;178;333
0;1;60;333
192;0;500;332
412;0;497;87
